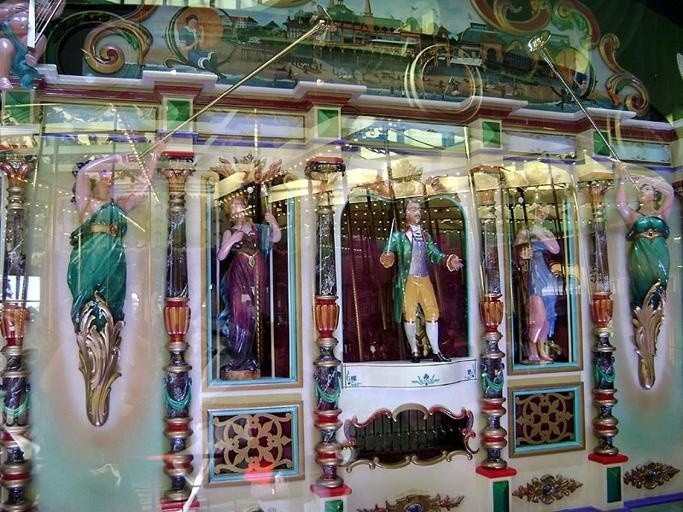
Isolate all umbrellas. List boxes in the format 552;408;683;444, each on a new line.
177;5;222;48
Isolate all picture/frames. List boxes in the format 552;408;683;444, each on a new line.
200;151;306;489
500;159;585;460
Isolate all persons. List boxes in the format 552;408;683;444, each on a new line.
614;164;674;313
178;14;226;79
379;199;464;362
64;140;165;337
214;192;283;371
512;202;562;363
267;56;538;99
0;0;67;90
